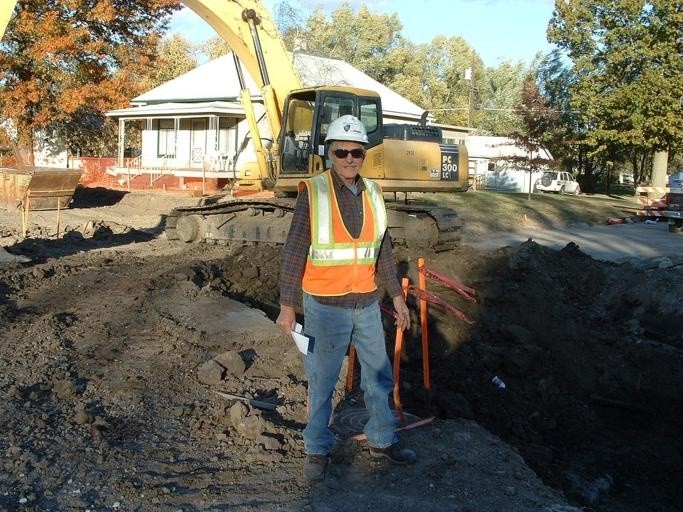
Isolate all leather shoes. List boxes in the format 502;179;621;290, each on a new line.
302;450;328;481
370;440;417;465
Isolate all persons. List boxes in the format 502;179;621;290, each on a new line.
275;113;418;482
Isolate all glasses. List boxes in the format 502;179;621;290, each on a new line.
332;148;363;159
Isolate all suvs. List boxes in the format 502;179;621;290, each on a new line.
534;170;580;196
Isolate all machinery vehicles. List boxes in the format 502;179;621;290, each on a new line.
160;0;473;258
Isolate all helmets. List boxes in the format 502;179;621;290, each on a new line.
324;114;369;145
